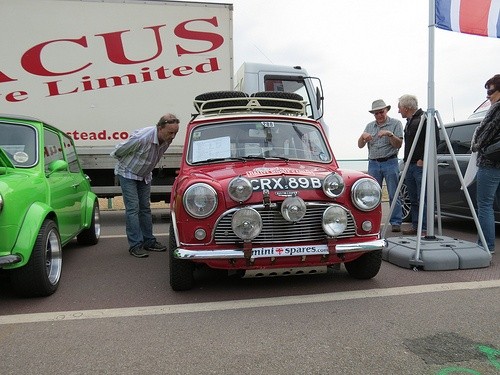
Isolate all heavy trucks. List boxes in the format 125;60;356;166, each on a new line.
0;0;330;204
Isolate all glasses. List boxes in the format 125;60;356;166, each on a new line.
487;88;498;95
159;119;179;126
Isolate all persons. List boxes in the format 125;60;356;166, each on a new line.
109;113;180;258
397;94;440;236
469;74;500;254
262;132;289;157
357;98;403;232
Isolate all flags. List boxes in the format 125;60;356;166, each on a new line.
435;0;500;39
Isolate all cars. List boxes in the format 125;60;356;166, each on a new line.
398;117;500;232
0;113;102;298
167;90;387;292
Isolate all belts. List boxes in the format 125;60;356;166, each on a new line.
371;154;397;162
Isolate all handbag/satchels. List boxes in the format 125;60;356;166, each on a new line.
460;151;480;190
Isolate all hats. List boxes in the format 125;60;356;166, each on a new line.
369;99;391;114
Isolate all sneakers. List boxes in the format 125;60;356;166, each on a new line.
129;246;149;258
402;226;417;234
421;231;427;236
392;225;401;232
146;241;167;252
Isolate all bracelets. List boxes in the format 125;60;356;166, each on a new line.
388;133;394;137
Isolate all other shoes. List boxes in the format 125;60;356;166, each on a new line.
489;250;495;254
